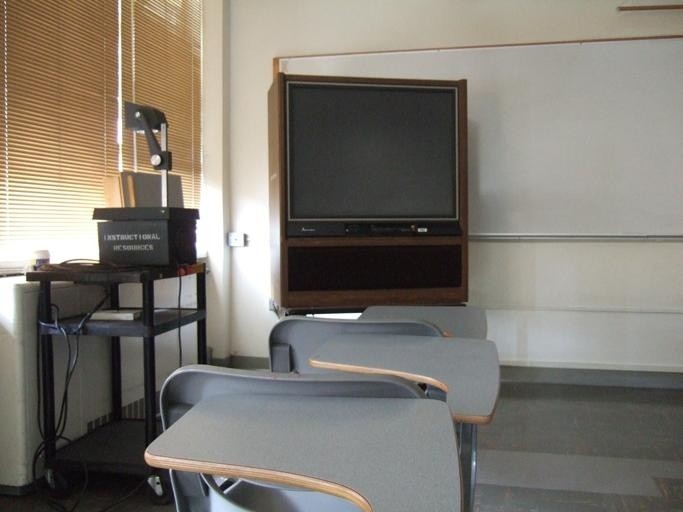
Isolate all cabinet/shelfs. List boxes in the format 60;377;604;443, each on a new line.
263;69;471;318
24;254;216;506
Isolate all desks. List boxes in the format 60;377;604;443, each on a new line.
303;333;508;508
138;390;468;512
355;305;490;338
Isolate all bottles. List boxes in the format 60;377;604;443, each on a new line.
34;250;49;271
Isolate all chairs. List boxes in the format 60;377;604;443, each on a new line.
264;315;450;375
152;359;430;512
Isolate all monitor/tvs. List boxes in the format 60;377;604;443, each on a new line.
286;81;462;238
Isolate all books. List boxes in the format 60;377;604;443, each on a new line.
87;307;143;321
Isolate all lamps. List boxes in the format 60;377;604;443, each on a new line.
121;97;175;209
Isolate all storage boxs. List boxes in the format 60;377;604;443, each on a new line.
91;204;202;267
